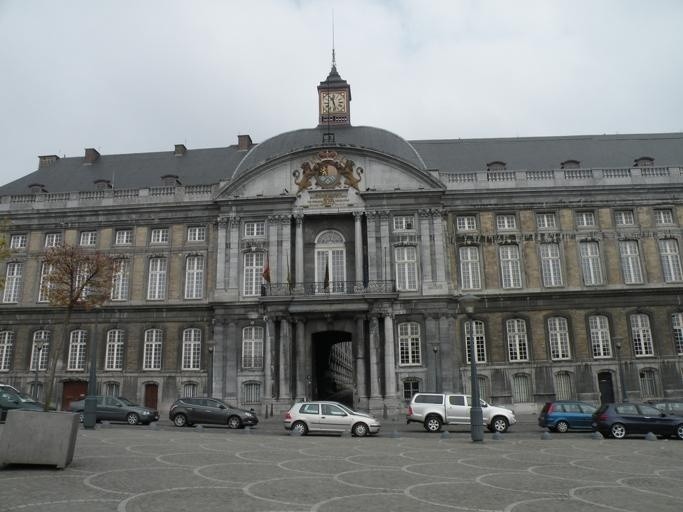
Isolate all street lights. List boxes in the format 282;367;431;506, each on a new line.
82;288;105;429
457;291;485;444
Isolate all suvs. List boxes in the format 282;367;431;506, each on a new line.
0;384;56;422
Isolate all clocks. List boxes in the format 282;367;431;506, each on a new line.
320;91;347;113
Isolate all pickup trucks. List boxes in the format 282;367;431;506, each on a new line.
408;391;516;434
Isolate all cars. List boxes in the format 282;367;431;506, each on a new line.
281;400;380;436
67;396;160;426
538;400;683;439
168;397;258;429
